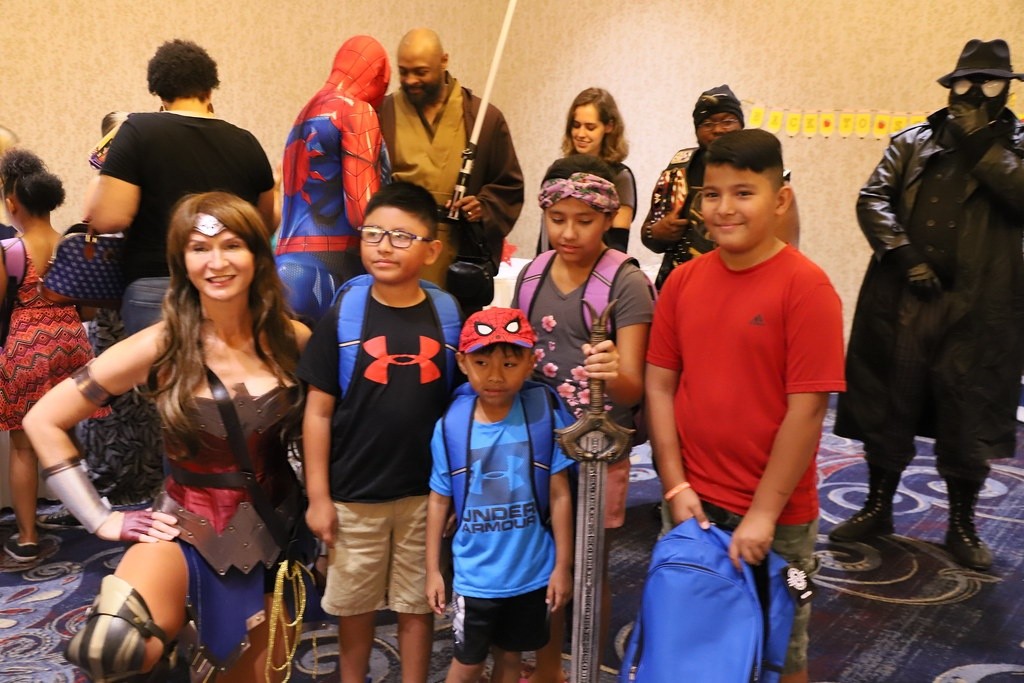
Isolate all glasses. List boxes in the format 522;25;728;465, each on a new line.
360;224;430;249
698;118;740;131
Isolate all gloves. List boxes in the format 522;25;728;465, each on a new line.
908;263;944;301
947;101;990;141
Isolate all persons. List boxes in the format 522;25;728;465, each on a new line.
639;84;800;298
421;308;577;683
3;27;526;557
537;84;636;253
509;152;654;683
298;181;469;682
642;128;847;682
829;34;1023;568
19;192;312;683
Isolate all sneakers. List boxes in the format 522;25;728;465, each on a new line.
4;532;41;562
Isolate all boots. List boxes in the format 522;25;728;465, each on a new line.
830;464;901;543
946;480;996;569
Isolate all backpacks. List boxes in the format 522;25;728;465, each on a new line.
621;518;799;683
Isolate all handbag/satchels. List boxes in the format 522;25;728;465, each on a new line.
37;222;126;309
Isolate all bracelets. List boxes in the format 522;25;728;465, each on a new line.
661;481;690;503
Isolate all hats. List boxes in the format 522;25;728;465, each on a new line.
937;39;1024;89
693;85;745;128
458;307;537;353
